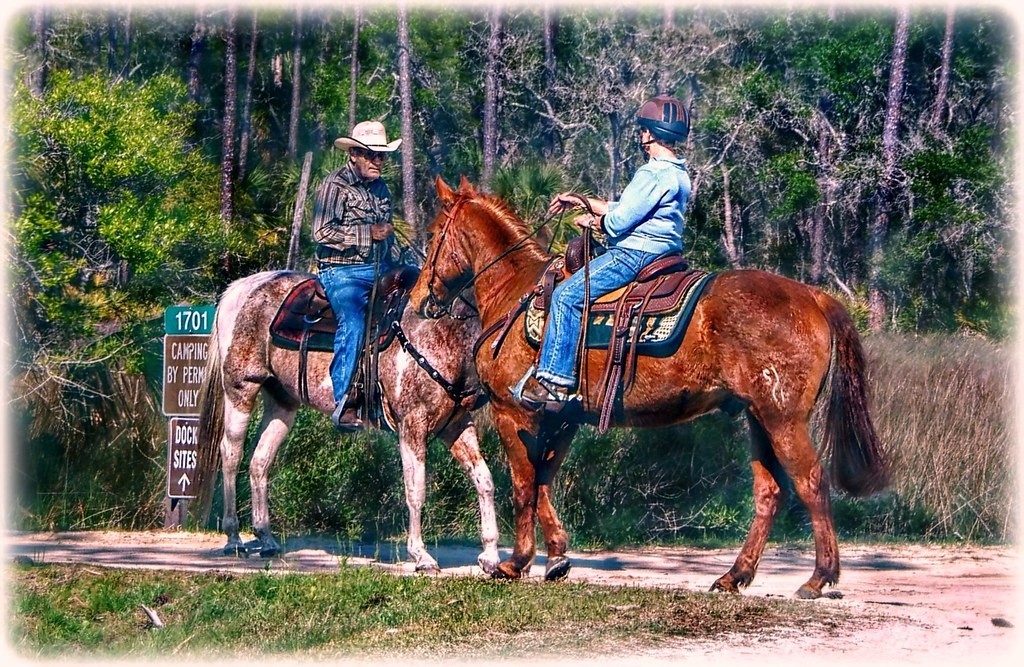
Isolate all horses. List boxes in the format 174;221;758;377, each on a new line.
410;173;887;600
191;225;547;574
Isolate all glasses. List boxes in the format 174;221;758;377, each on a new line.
358;152;389;161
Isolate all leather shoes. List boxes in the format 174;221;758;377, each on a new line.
335;407;364;430
508;379;570;415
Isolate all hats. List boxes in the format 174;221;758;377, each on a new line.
334;120;402;151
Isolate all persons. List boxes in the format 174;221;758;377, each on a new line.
312;120;421;429
507;94;692;413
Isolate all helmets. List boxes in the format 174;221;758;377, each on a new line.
636;96;690;143
